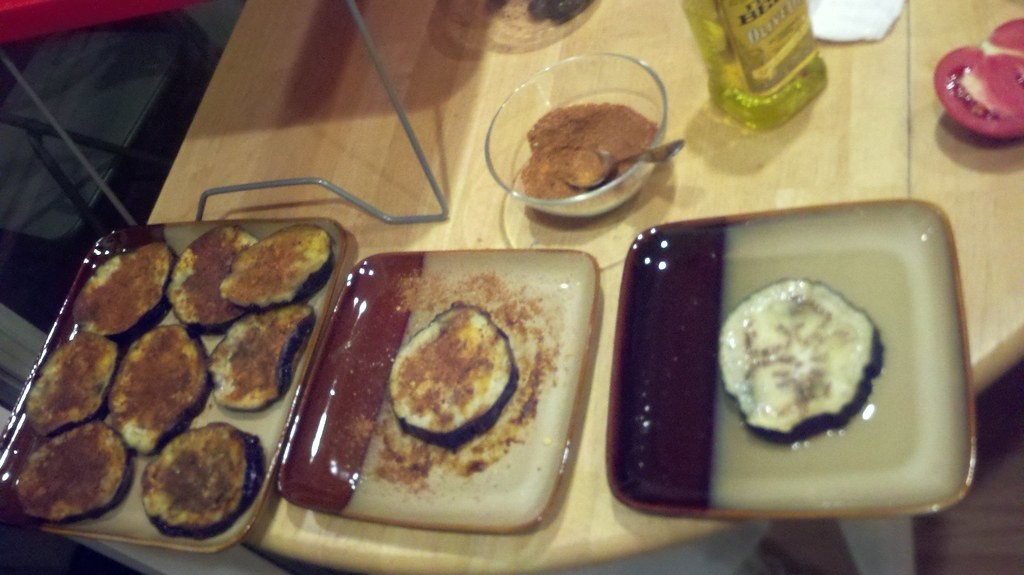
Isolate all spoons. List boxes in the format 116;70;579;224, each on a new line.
564;140;687;189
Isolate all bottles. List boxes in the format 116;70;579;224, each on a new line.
677;0;829;133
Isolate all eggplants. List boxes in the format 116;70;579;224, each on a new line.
718;276;883;440
390;304;516;448
16;223;336;539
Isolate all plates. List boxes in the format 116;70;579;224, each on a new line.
605;200;977;520
1;215;348;555
275;250;601;537
443;1;603;56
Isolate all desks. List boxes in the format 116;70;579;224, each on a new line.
68;0;1024;574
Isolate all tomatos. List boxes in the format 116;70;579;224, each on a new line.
934;16;1024;139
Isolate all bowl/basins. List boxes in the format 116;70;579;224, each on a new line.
484;52;669;218
